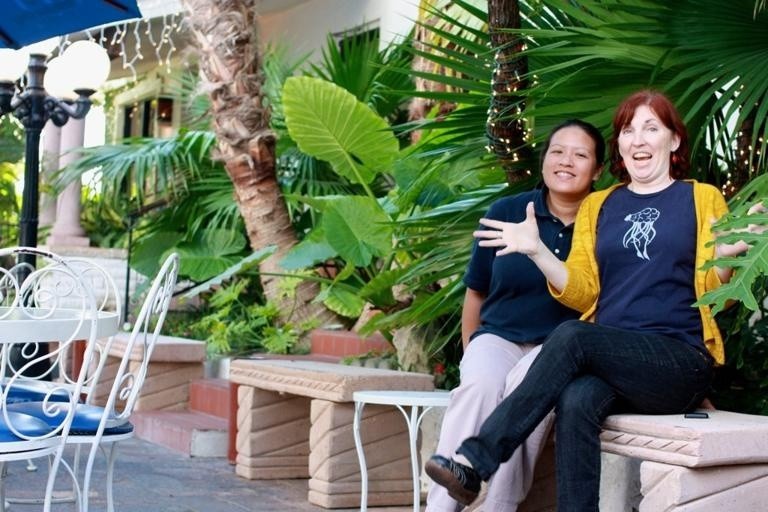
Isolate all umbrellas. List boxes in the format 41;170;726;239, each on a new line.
0;1;189;53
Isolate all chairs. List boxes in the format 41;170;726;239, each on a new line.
4;252;179;512
1;255;121;477
0;247;98;511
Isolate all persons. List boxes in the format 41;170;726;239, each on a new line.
425;117;717;511
423;86;768;511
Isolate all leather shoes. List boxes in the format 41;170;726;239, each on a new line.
424;455;482;506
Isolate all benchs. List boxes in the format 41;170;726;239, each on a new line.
87;333;207;412
229;360;434;509
598;411;767;512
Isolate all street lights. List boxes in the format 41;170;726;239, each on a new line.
1;37;112;382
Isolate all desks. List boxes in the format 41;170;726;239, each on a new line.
351;391;452;512
1;307;118;506
